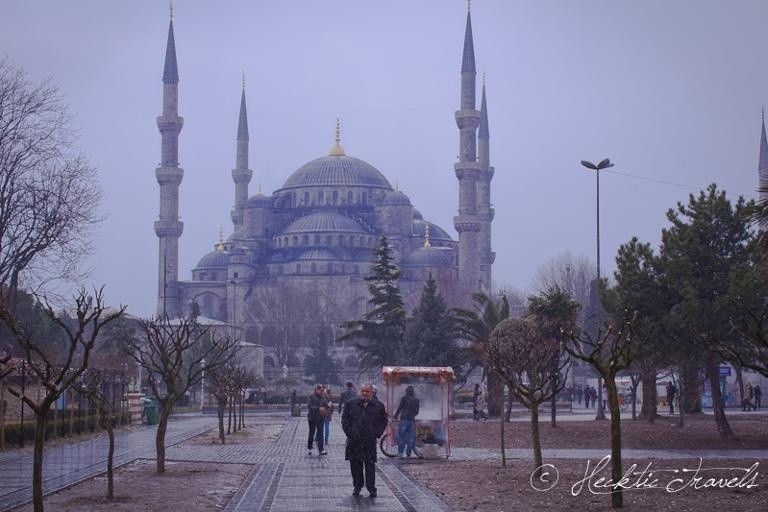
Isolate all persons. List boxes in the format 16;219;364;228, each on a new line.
306;384;327;455
559;381;608;410
474;387;488;421
666;381;675;415
742;382;757;411
471;383;480;420
341;382;387;498
754;384;763;408
316;388;334;446
747;381;754;410
391;384;420;459
337;381;360;413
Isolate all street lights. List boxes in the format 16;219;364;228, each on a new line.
581;158;615;419
226;280;236;340
200;358;207;409
164;248;172;316
13;360;35;448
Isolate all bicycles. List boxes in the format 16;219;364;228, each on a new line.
380;416;441;458
